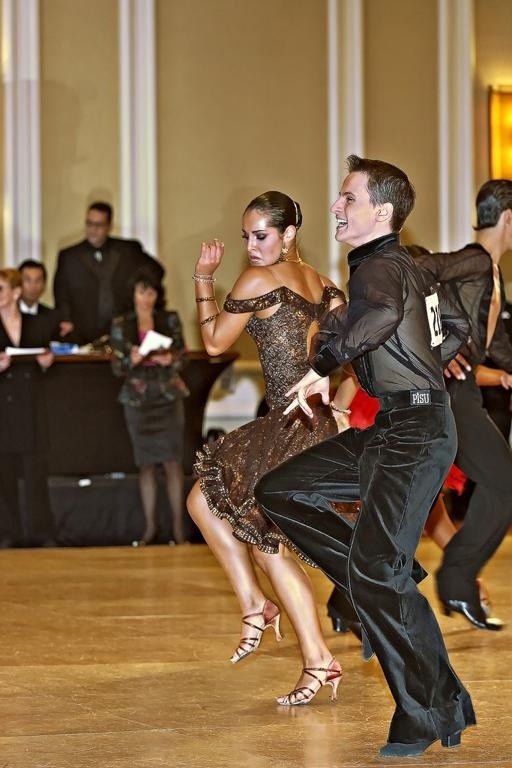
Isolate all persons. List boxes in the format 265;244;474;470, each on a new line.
453;301;511;519
192;277;216;283
187;191;357;711
253;156;478;757
52;202;143;345
16;260;56;339
111;273;191;545
0;269;54;545
416;178;511;630
331;246;491;608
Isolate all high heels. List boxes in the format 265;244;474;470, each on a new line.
332;617;362;642
379;730;461;757
275;656;341;707
231;601;283;664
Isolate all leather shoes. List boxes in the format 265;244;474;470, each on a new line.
441;590;503;631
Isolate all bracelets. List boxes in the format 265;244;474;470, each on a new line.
500;372;508;391
343;368;355;377
200;313;220;328
329;400;351;415
195;275;214;278
195;296;215;302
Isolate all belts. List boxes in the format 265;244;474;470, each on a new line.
378;389;451;412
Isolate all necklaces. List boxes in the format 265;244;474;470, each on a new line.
279;259;302;264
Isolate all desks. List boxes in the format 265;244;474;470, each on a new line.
38;344;242;474
